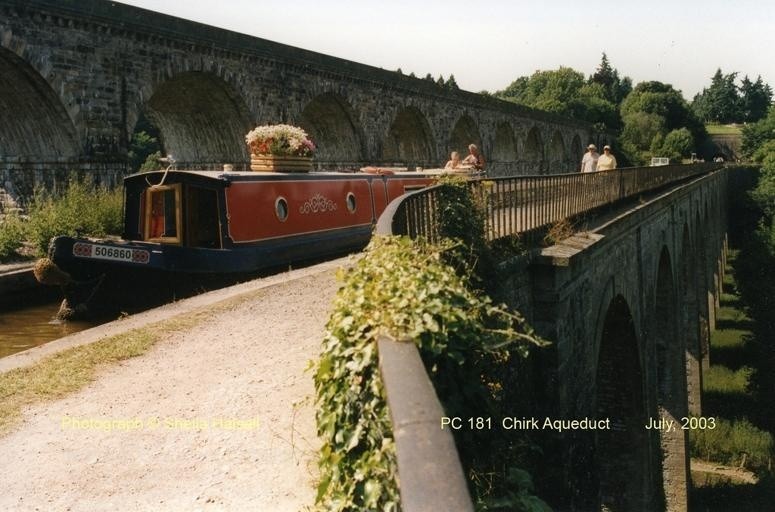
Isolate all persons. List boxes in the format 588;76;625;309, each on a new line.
595;145;618;173
444;151;462;170
463;144;485;171
581;143;601;174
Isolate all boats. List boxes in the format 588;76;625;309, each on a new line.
33;154;498;320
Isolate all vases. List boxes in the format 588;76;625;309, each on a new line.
249;153;315;173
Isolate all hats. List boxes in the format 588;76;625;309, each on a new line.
586;144;597;150
604;144;611;150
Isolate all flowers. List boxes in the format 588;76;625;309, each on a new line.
245;123;318;158
